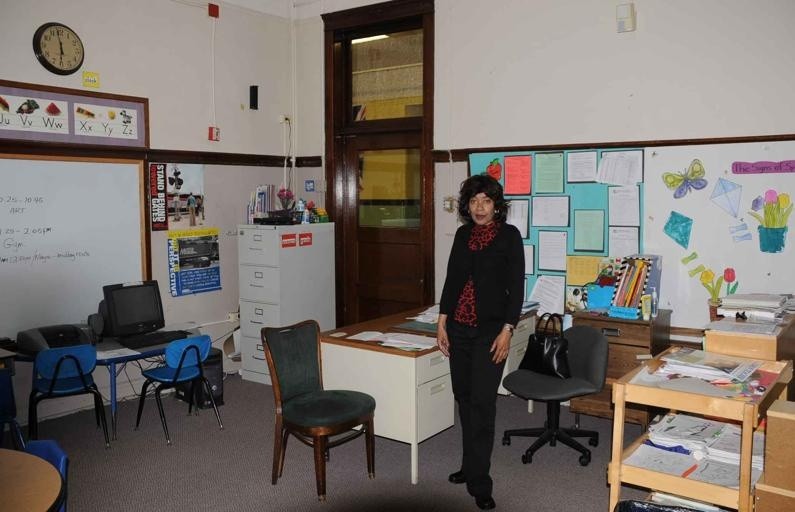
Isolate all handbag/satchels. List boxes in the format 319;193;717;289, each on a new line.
518;312;570;380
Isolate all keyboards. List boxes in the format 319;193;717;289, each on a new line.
121;332;187;349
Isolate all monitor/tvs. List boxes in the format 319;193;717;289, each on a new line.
99;281;164;336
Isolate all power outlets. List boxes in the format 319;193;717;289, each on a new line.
280;115;289;123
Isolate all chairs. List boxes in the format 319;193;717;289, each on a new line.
26;439;69;512
135;335;224;447
28;344;110;448
503;326;609;466
0;367;26;451
261;320;376;504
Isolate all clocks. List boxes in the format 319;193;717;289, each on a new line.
33;22;84;75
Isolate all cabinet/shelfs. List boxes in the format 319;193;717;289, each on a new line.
608;344;794;512
569;309;672;436
237;222;336;385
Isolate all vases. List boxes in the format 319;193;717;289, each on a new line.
758;225;788;253
707;298;725;322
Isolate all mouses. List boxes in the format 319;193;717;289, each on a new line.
177;330;184;333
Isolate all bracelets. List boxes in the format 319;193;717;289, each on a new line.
502;325;513;337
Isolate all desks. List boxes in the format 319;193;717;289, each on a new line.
320;304;537;485
704;314;795;360
0;448;64;512
15;332;203;441
0;349;17;372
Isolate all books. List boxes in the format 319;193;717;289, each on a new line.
659;346;764;384
255;183;274;214
599;256;653;309
716;296;795;324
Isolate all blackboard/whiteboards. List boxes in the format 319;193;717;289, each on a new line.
0;153;147;343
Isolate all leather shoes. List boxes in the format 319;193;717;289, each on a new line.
474;494;496;510
449;471;467;484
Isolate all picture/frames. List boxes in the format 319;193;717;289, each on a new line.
0;79;150;151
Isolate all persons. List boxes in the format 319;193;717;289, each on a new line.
567;288;588;312
186;192;197;226
437;173;524;511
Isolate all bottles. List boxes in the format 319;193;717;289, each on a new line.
297;200;310;224
641;294;653;323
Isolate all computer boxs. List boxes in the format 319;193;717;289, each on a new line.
183;348;223;409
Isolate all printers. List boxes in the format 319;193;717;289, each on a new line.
17;324;91;358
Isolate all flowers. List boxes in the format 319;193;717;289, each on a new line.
700;269;723;303
746;189;793;228
724;268;738;295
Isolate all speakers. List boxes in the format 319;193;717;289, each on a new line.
88;314;105;342
